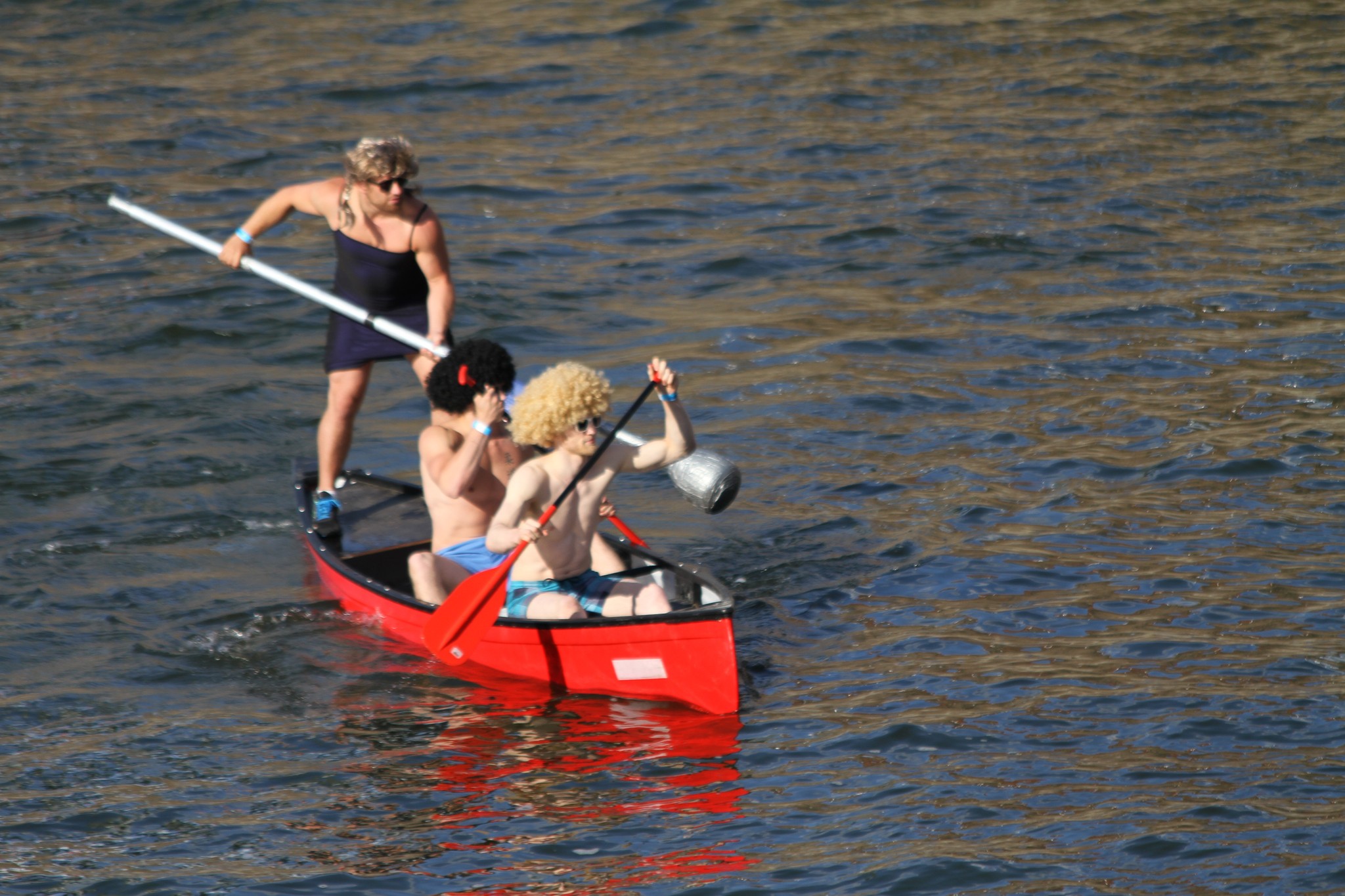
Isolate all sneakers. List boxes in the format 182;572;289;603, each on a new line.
310;490;344;541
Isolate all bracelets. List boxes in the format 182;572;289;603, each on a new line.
235;227;254;244
657;391;678;402
472;421;492;436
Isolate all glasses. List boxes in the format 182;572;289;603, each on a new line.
366;173;408;193
574;414;602;432
493;383;510;394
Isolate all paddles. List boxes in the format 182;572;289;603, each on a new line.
421;364;662;667
458;365;652;549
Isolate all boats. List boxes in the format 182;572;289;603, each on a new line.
293;466;742;716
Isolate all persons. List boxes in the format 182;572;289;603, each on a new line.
218;140;455;538
407;342;696;619
